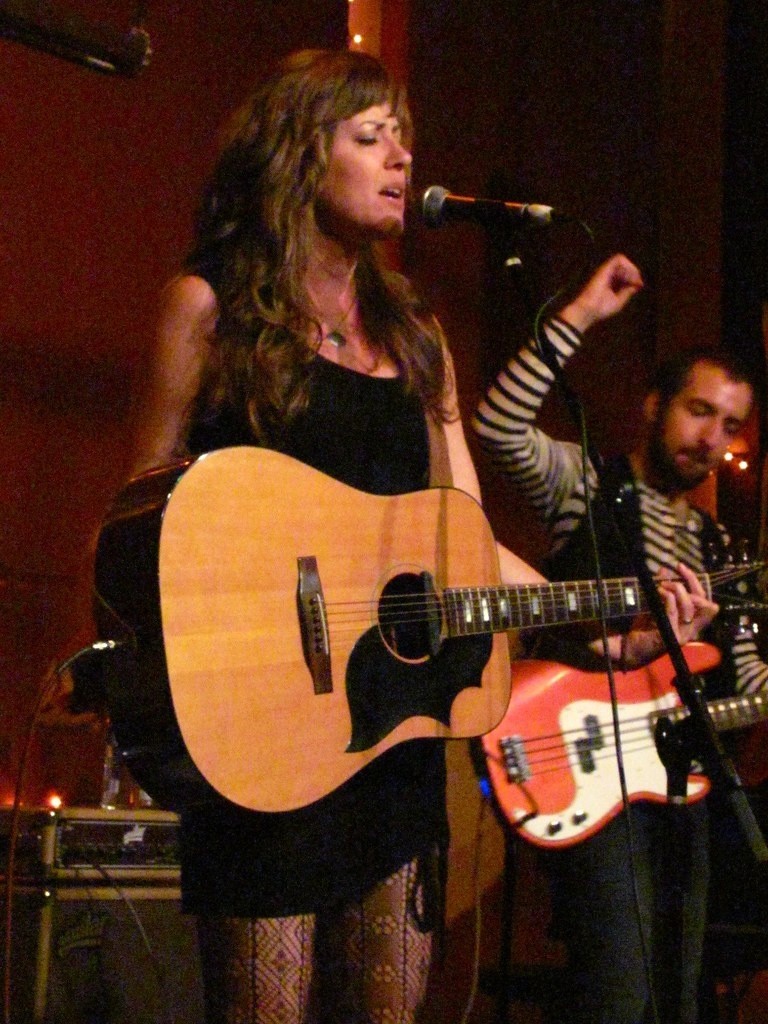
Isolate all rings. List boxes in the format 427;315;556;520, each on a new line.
681;618;693;624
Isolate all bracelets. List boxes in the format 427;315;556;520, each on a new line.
622;633;627;668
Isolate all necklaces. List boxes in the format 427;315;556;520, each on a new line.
327;299;355;346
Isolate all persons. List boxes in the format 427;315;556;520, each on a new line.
470;253;768;1023
127;50;720;1023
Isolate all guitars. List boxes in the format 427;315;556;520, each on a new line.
470;641;767;850
95;442;713;827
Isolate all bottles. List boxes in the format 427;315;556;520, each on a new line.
101;746;154;809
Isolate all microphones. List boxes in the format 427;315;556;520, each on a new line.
421;185;552;228
